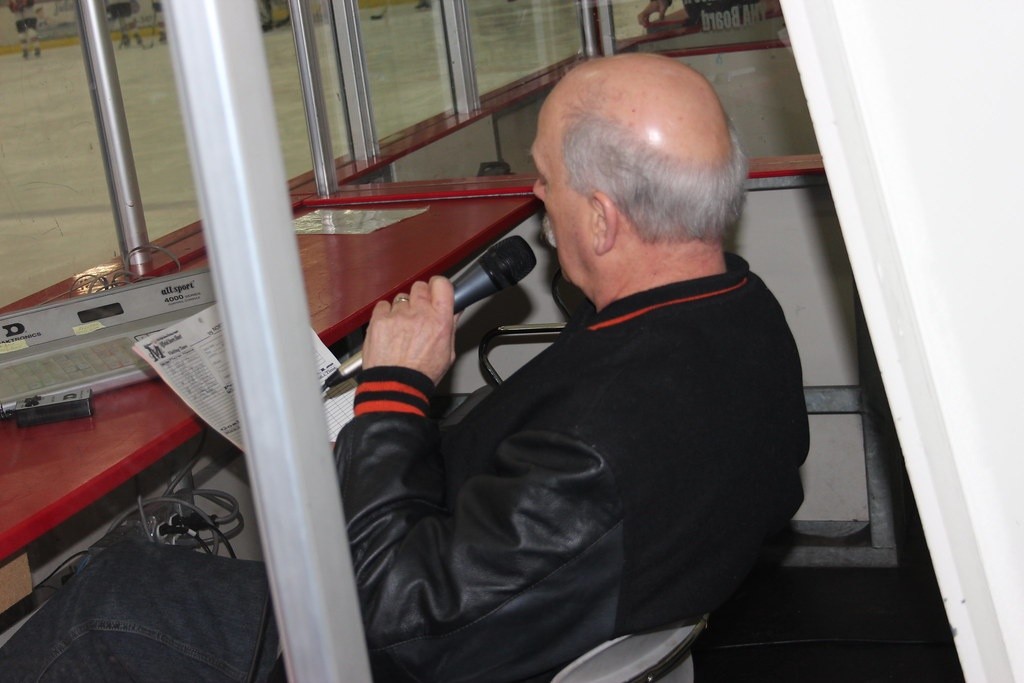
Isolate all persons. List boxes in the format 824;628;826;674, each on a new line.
8;1;297;60
639;0;761;30
0;52;810;683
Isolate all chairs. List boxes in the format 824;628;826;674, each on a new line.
550;611;715;682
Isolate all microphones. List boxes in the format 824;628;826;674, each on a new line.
325;234;536;389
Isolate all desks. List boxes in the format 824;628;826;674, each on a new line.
0;196;535;563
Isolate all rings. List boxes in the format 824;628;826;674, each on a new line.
393;295;410;304
